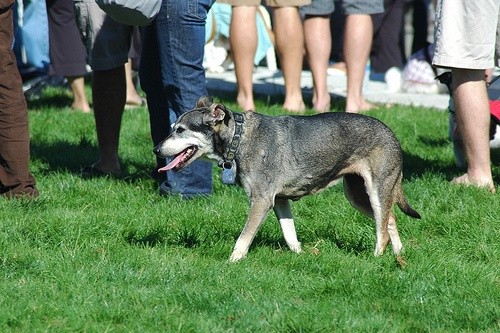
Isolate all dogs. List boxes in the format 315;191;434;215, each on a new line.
151;95;422;262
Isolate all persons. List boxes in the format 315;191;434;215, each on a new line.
138;0;216;198
80;0;136;183
11;0;436;116
0;0;41;199
430;0;500;192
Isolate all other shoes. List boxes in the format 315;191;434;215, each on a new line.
76;166;106;180
